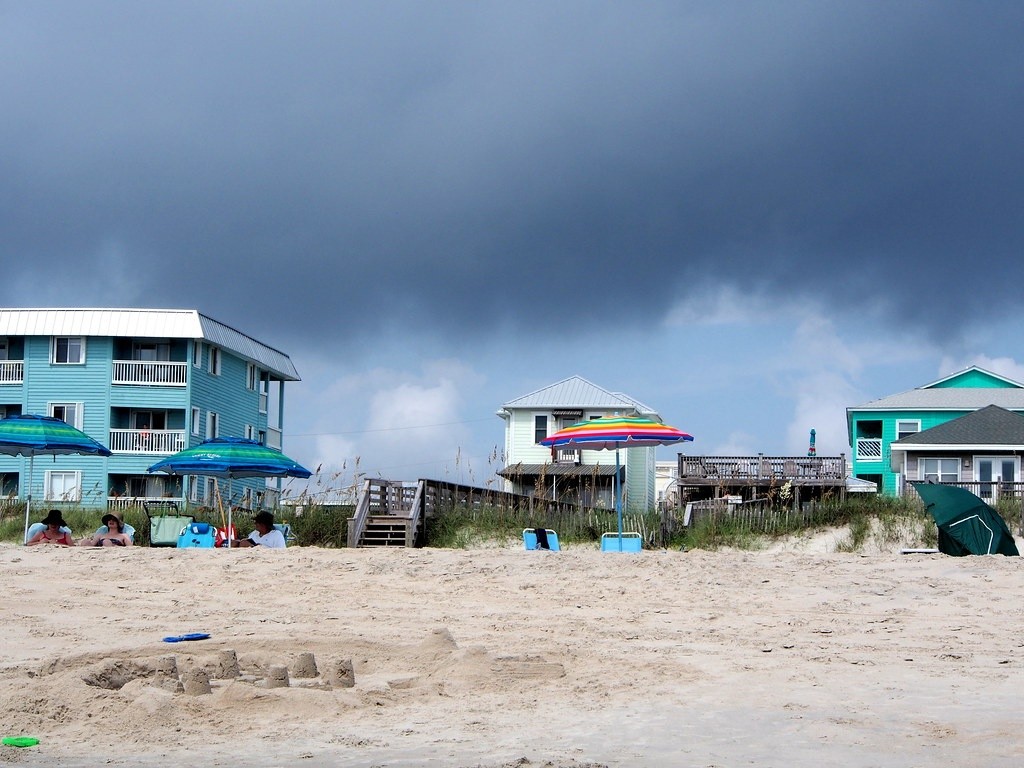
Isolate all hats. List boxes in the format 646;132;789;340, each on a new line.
251;511;274;526
41;510;67;526
101;511;124;530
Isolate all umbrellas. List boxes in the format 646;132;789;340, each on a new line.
538;411;694;552
146;435;313;549
807;429;817;456
909;484;1020;557
0;414;114;545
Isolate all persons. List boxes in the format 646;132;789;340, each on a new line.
24;510;76;548
231;510;286;549
81;511;133;547
138;424;150;451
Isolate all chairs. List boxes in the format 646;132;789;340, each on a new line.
758;460;772;477
272;524;291;542
601;531;643;553
94;522;137;540
783;460;799;479
27;522;72;542
733;460;751;476
176;522;217;548
523;527;561;552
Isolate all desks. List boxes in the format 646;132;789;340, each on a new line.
798;463;822;479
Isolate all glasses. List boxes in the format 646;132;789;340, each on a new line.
48;521;60;525
106;517;118;522
